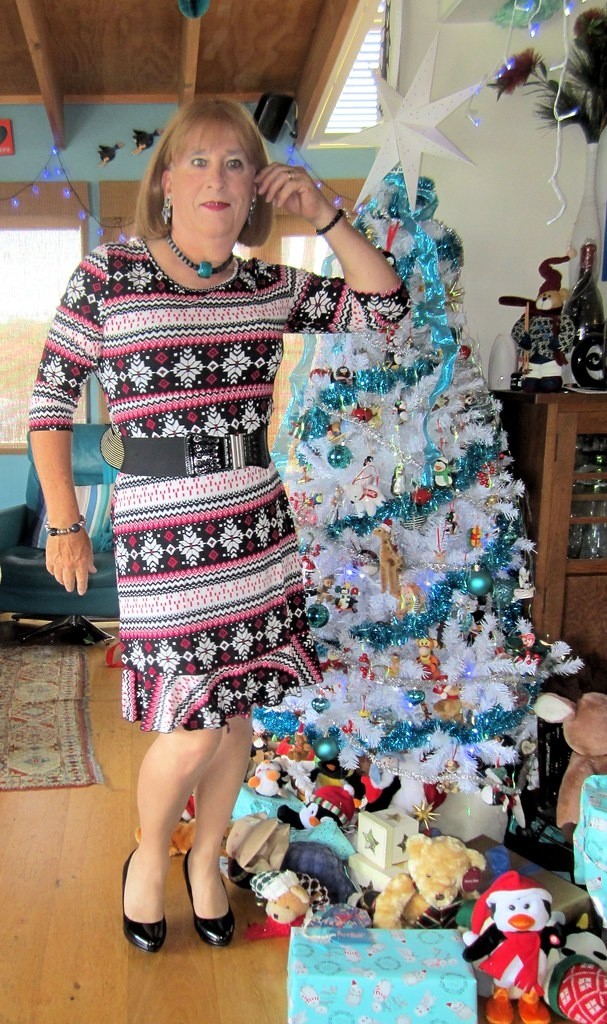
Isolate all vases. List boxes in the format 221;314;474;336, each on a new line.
568;142;603;294
488;333;519;391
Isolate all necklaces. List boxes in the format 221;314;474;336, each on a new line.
166;230;234;277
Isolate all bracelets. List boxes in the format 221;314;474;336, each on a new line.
44;514;87;536
316;208;344;235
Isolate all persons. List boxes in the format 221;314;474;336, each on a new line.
28;96;413;953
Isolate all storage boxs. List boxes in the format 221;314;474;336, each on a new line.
231;777;595;1024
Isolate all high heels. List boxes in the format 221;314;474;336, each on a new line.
182;847;235;947
122;847;167;953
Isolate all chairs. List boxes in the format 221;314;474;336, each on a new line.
0;422;130;645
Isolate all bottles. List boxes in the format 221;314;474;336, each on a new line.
565;244;604;333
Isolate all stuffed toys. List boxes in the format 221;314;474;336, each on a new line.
373;833;485;929
135;723;447;933
250;247;541;810
534;693;607;840
462;871;607;1024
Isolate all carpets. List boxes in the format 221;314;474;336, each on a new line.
0;644;106;792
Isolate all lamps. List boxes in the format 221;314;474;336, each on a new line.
255;91;299;144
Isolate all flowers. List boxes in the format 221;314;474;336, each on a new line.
481;5;607;148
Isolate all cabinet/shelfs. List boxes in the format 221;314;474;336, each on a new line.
488;387;607;701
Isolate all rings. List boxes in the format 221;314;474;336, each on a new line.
288;170;292;180
